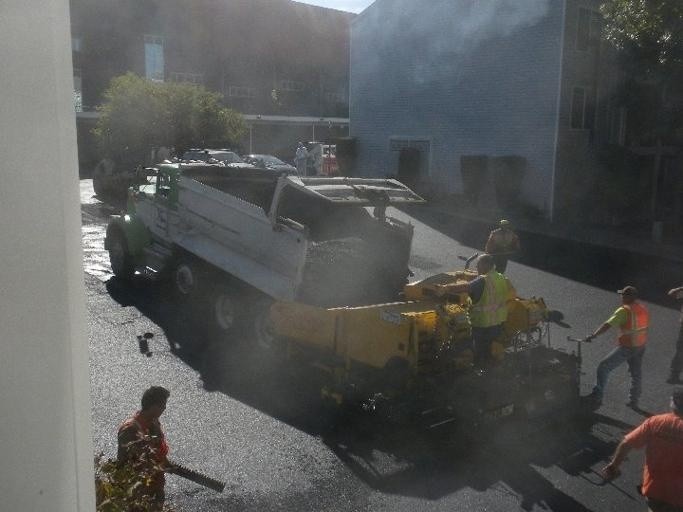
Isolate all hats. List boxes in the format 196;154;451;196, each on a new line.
669;386;683;410
616;285;640;298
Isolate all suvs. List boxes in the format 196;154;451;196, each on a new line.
179;147;246;164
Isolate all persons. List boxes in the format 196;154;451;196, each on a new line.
485;218;520;273
117;385;171;512
293;141;310;175
434;252;517;338
586;284;650;409
665;285;683;383
603;387;683;512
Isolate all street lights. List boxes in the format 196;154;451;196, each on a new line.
312;117;324;141
249;114;261;154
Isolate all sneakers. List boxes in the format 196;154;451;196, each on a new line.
665;370;679;384
623;398;639;412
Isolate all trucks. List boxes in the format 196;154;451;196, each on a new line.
104;160;427;351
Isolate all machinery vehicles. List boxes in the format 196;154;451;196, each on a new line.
254;270;588;458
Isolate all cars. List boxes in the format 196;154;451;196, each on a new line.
244;154;296;175
